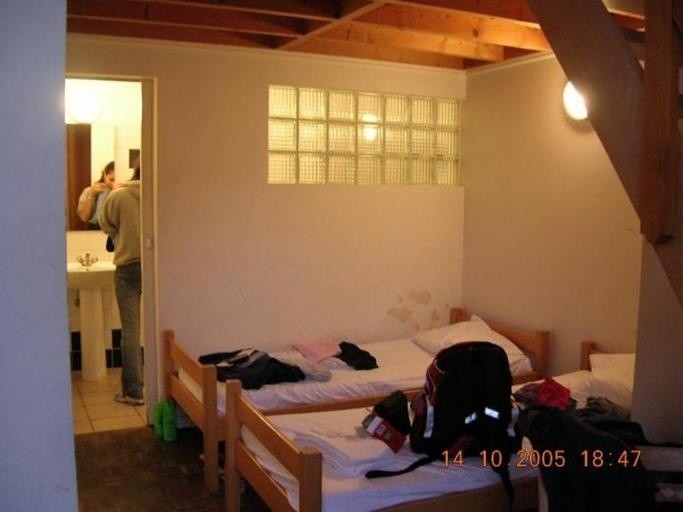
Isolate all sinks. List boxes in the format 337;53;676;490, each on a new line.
67;261;117;290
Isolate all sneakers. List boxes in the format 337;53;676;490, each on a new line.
111;389;146;407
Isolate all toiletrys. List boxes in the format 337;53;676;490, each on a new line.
153;402;164;440
162;402;177;441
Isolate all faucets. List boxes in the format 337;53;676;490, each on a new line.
84;251;91;266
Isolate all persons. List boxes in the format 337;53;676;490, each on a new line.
76;162;114;230
96;156;144;407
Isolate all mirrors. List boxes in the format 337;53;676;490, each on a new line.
66;117;120;230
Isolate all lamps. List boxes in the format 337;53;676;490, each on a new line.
562;81;587;121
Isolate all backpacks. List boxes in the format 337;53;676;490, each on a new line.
408;340;513;470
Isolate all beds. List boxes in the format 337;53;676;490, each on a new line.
226;339;601;512
162;307;549;491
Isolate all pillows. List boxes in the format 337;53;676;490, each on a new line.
412;314;533;376
589;353;635;407
